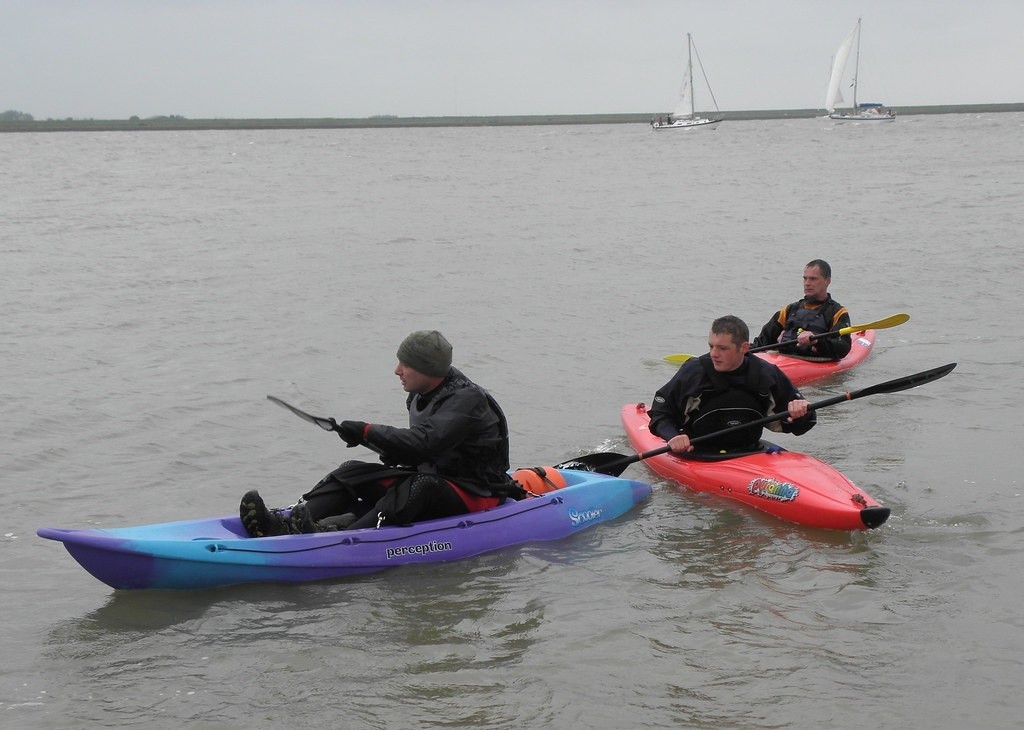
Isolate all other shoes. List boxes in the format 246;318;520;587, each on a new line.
239;489;290;537
288;504;326;535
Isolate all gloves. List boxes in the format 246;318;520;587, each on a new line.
338;420;364;448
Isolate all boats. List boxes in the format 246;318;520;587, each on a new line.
622;403;893;530
36;465;644;589
753;331;878;386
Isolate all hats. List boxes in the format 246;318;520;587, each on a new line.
396;329;453;377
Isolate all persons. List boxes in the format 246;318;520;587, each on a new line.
658;117;663;126
647;315;817;454
239;329;511;537
877;107;881;113
666;114;671;125
649;115;654;124
888;109;891;115
749;259;852;359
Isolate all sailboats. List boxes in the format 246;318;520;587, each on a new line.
825;17;896;122
650;32;725;132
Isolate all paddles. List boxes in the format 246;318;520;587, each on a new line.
558;362;959;478
266;394;383;458
663;311;909;363
